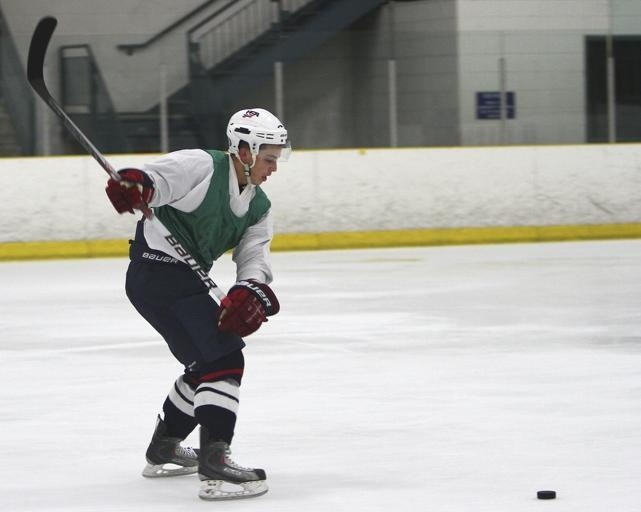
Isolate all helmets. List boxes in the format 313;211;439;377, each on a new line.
225;108;287;168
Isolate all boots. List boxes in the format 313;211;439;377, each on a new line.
146;415;200;468
196;427;266;481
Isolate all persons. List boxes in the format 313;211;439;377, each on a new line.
105;106;288;484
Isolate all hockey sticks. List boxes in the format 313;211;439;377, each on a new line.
27;17;231;307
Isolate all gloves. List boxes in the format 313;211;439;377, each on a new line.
106;169;153;213
216;280;280;337
